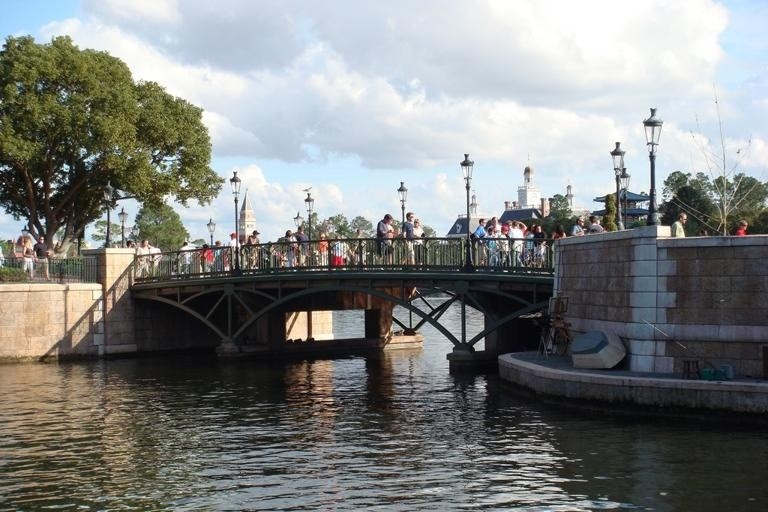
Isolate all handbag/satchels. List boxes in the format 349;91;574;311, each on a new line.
470;234;477;242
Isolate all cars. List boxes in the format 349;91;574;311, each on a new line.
0;246;36;268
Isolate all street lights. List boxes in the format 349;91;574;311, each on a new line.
644;108;664;226
132;225;140;246
118;207;128;248
21;226;28;238
460;154;480;274
206;219;216;245
231;172;245;277
305;193;314;249
610;143;631;230
398;182;407;223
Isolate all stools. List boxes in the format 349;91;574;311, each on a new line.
682;356;701;379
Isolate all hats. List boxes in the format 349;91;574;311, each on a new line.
501;226;509;231
229;233;236;237
215;241;221;244
253;230;260;234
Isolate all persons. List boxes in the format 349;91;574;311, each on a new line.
671;212;688;237
472;215;605;273
21;238;33;282
734;220;748;236
110;214;426;285
32;236;51;282
